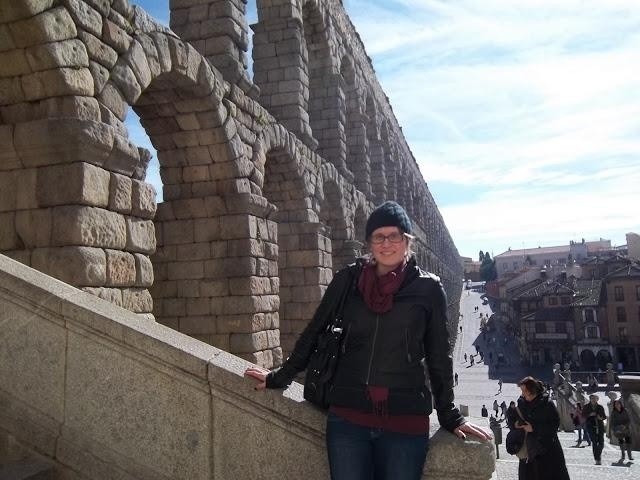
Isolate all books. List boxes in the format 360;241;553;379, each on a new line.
507;407;529;426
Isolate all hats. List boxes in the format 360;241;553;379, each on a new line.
365;200;413;242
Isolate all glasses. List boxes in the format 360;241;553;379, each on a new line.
370;232;412;244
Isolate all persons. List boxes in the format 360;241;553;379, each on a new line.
575;401;591;445
454;305;515;431
507;376;571;480
244;199;494;480
609;401;635;461
582;394;606;465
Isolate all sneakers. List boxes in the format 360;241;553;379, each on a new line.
576;442;591;447
595;458;601;465
621;457;635;461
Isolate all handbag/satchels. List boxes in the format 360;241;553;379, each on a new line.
506;404;530;464
303;260;363;409
